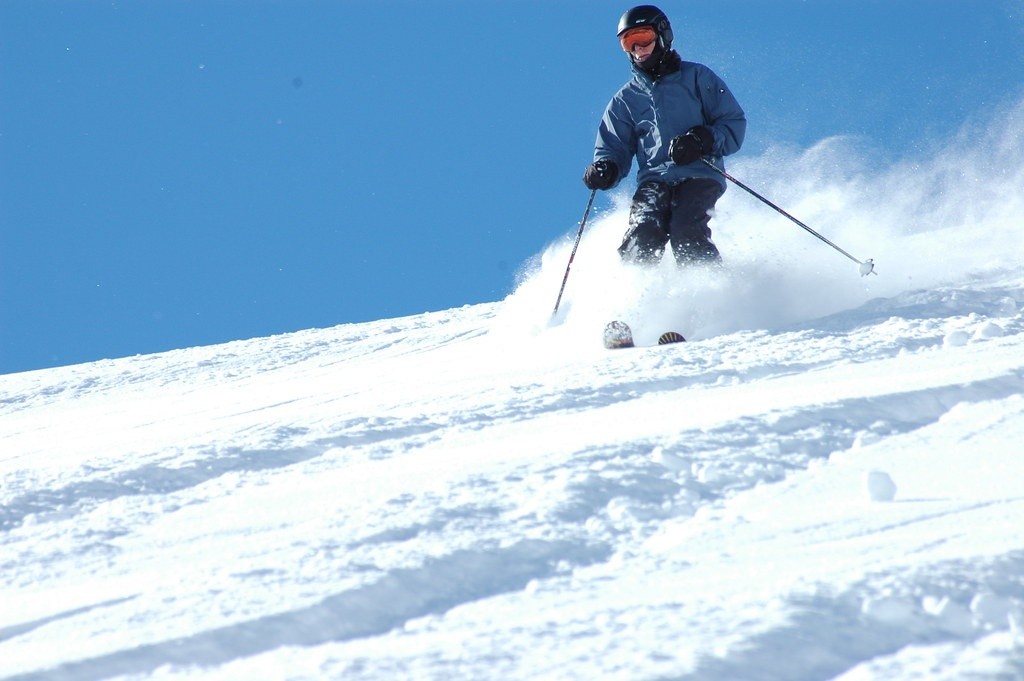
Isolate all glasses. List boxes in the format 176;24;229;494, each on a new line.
620;28;656;52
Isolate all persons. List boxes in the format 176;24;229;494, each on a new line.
583;4;747;266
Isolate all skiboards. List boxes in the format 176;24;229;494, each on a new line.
593;319;707;348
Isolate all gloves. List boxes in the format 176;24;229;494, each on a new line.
668;125;715;166
584;160;617;191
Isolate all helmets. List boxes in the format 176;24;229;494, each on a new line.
616;5;673;70
